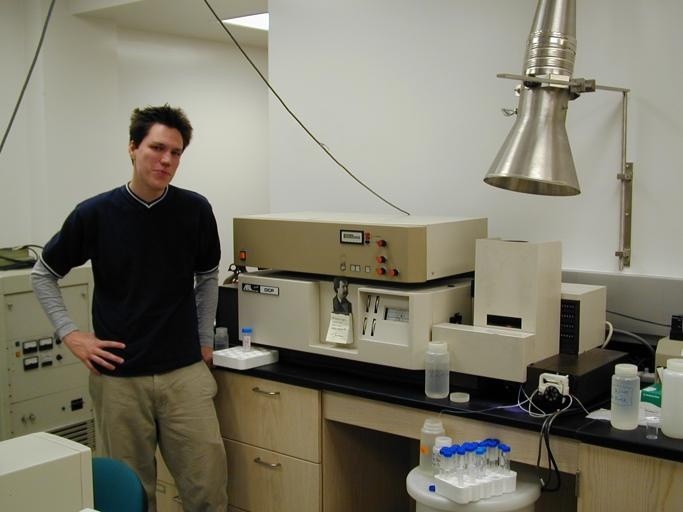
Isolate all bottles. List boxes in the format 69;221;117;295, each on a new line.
419;418;512;487
609;363;641;431
214;327;229;351
241;327;253;352
424;340;451;400
659;358;683;440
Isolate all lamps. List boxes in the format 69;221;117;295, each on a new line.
484;0;634;271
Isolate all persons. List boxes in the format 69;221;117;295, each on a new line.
331;277;352;315
28;102;231;512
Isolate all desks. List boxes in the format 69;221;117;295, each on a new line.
209;342;682;511
